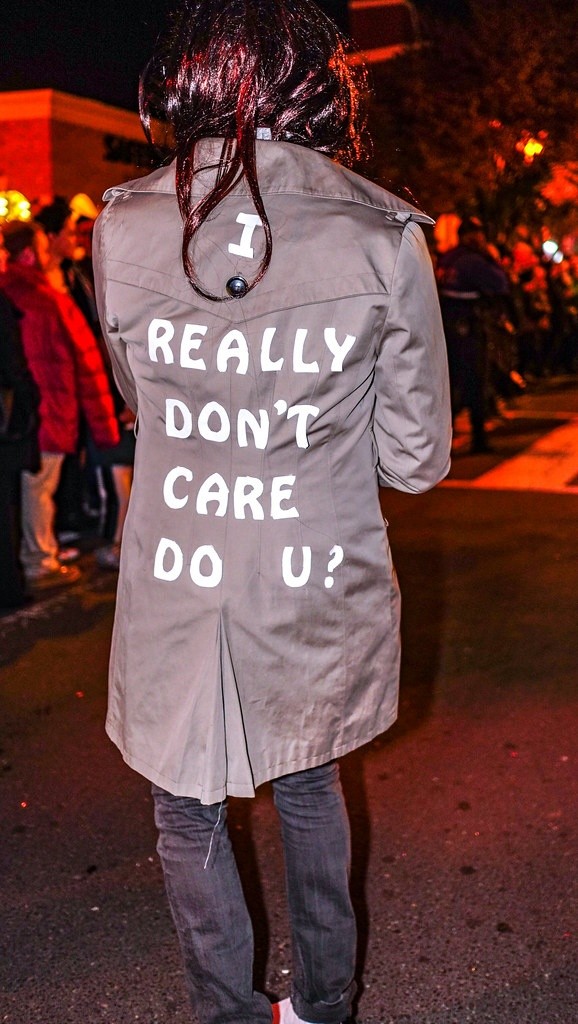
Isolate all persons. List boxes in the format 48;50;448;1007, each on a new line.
91;0;454;1024
0;194;578;614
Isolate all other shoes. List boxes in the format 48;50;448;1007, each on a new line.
272;996;311;1024
25;564;82;592
58;531;80;543
56;548;80;564
95;545;121;569
